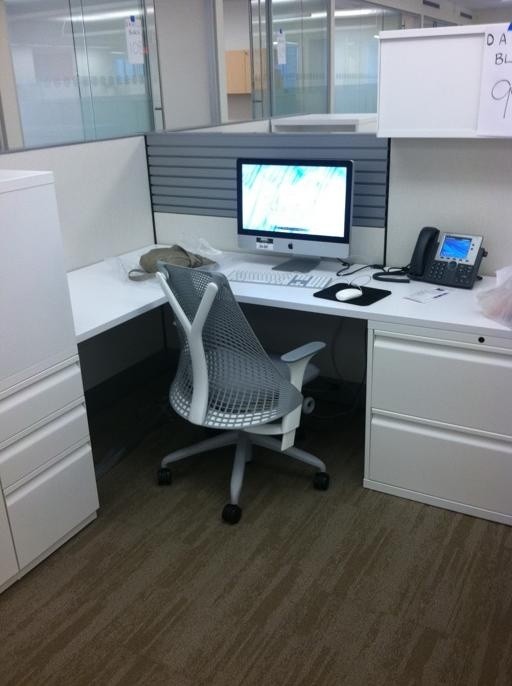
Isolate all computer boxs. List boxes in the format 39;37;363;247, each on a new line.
302;376;341;412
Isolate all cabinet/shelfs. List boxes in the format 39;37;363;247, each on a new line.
0;171;102;595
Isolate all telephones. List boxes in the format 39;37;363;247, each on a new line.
373;226;488;289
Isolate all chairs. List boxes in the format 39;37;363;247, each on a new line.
154;259;330;523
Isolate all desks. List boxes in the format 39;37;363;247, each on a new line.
65;242;510;525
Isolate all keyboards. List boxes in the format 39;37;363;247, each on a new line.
225;266;332;289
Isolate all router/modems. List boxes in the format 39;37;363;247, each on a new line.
325;402;356;421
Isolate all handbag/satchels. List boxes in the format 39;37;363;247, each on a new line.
128;245;220;281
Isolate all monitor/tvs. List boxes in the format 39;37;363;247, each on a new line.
236;157;354;273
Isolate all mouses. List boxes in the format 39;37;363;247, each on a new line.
336;288;362;301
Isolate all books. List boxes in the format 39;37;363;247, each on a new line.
274;111;378;124
274;123;378;132
